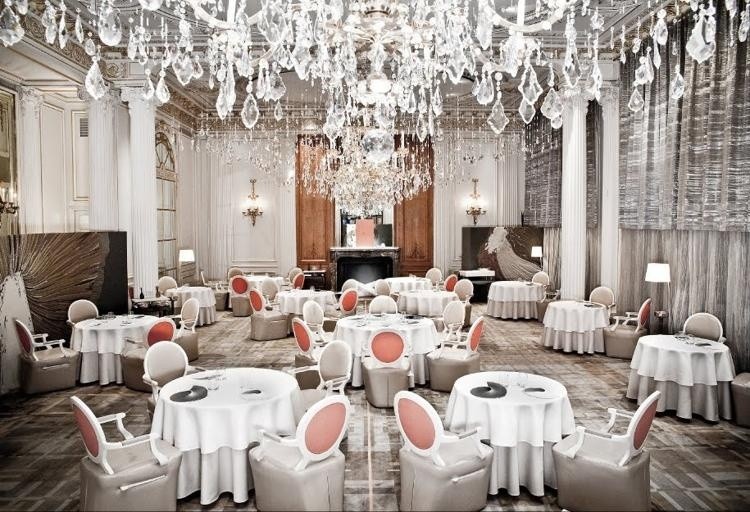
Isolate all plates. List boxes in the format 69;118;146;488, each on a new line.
303;270;326;291
460;269;495;303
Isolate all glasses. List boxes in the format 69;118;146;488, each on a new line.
0;189;19;227
0;0;750;218
465;180;488;226
531;246;543;270
645;263;671;284
242;179;264;227
178;249;194;262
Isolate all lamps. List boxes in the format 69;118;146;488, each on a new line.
142;341;189;423
15;319;79;394
342;279;357;291
375;278;390;295
121;317;176;393
322;288;358;332
369;294;397;316
228;268;243;281
552;390;662;512
454;279;474;329
303;300;332;347
444;274;456;292
730;372;750;426
248;288;287;341
231;275;251;316
435;302;466;346
293;272;305;289
158;276;177;294
425;316;484;392
536;287;562;322
248;395;351;512
360;327;409;408
425;268;443;289
201;270;228;310
164;297;200;362
292;318;320;391
603;297;652;359
589;286;617;317
532;271;550;289
69;396;183;511
394;390;494;512
683;313;723;343
280;268;302;288
291;340;352;441
66;299;99;327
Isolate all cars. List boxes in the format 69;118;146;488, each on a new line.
108;312;114;321
401;311;406;325
251;272;254;277
265;273;268;279
689;336;695;350
216;367;226;389
380;311;386;321
310;286;314;294
128;311;134;320
518;372;528;387
500;374;509;388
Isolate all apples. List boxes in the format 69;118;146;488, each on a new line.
2;188;5;201
8;187;13;202
14;194;18;207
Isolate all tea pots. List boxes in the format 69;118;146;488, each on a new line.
340;207;394;247
